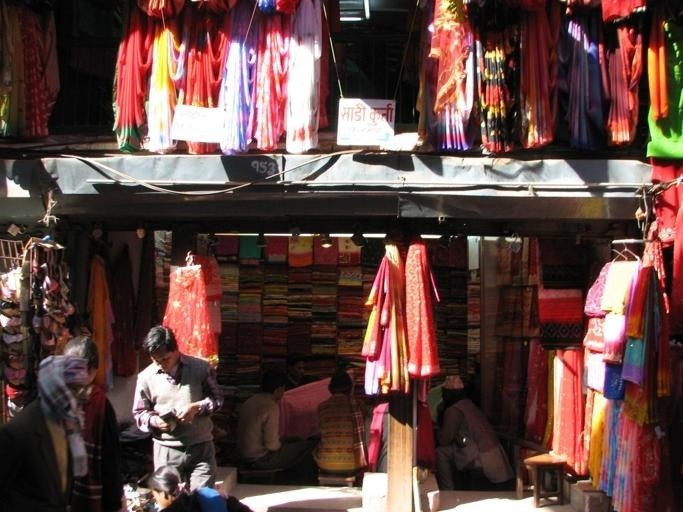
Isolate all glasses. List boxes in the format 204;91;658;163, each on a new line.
67;384;92;397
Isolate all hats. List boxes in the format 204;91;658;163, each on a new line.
442;374;463;390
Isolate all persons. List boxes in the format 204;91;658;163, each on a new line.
239;376;317;473
61;336;121;512
0;355;91;512
145;466;253;512
311;370;368;478
132;325;225;488
284;353;310;391
436;374;515;492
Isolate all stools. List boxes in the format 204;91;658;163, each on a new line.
318;475;356;487
516;456;562;506
239;468;285;483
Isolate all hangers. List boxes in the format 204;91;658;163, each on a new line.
185;250;196;266
609;240;641;262
